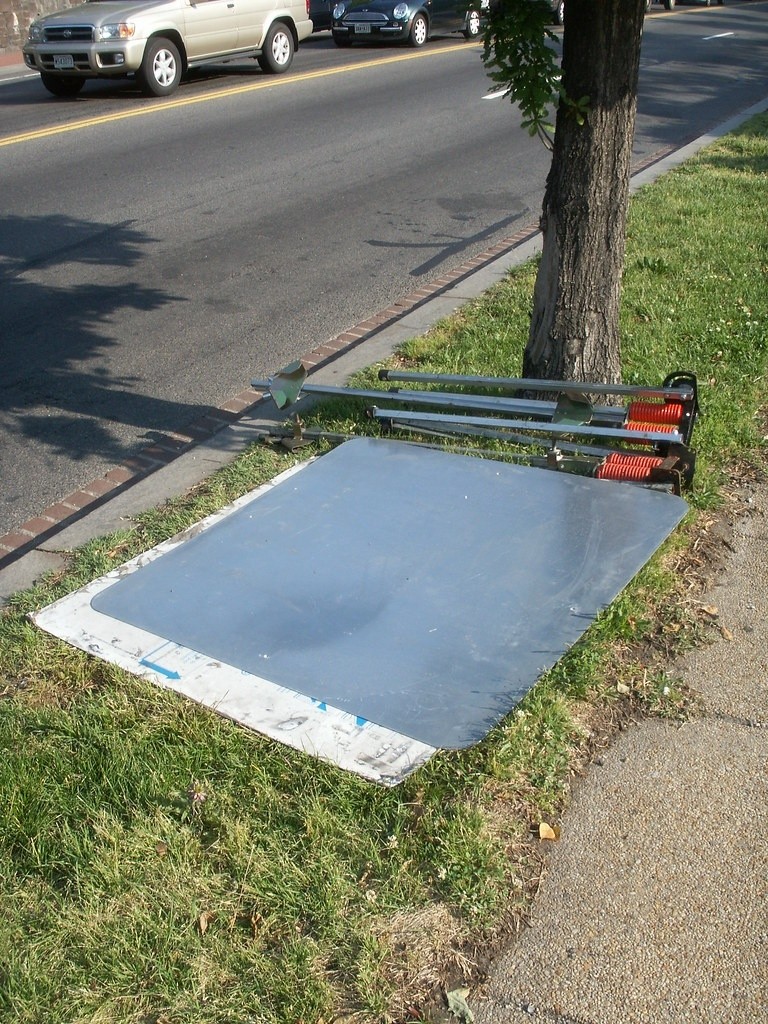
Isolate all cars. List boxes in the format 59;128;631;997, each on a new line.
306;0;490;48
21;0;315;98
